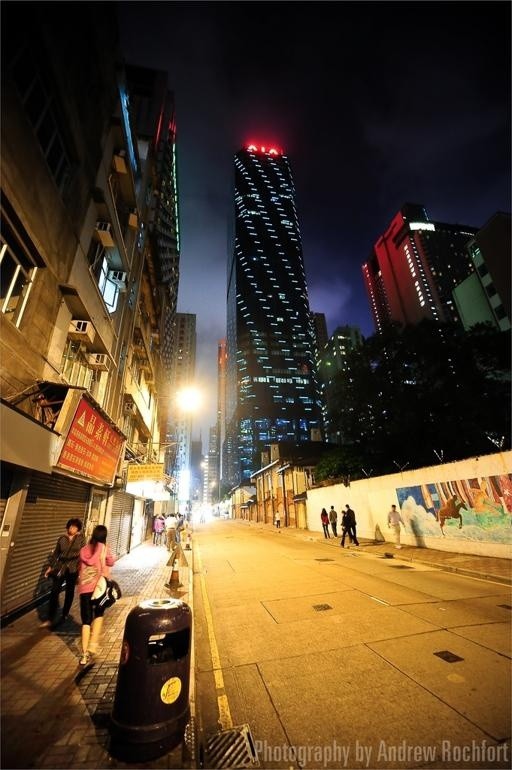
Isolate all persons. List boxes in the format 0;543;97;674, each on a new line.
151;512;183;552
340;510;359;547
321;509;330;539
387;505;405;549
345;504;356;542
44;518;86;621
275;511;280;528
76;525;114;665
329;506;338;538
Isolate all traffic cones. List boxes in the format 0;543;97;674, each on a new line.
165;553;182;588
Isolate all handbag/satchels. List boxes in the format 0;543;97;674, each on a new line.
47;563;64;582
89;543;122;611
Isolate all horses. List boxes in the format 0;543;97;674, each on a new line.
436;502;467;535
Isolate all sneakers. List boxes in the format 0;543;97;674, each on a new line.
394;543;403;550
325;536;359;549
37;620;53;629
87;644;99;657
152;543;176;552
78;650;90;666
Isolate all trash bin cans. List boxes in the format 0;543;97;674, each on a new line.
109;599;192;763
